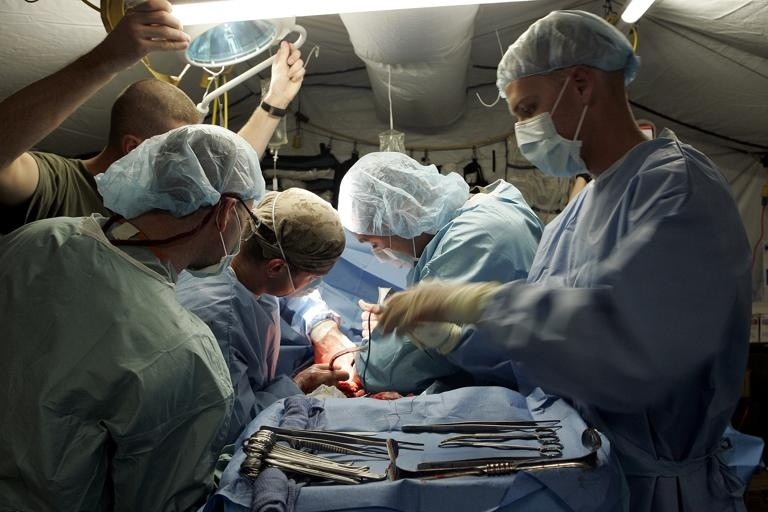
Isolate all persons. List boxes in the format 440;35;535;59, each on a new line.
162;180;361;446
0;125;266;510
365;9;753;511
335;149;544;395
0;0;307;231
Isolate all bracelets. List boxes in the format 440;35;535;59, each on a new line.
260;101;287;117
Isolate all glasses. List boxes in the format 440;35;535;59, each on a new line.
221;187;263;245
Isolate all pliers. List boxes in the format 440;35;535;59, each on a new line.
438;427;564;458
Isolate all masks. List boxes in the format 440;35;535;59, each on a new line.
274;270;325;301
183;197;248;280
366;213;424;269
514;67;595;182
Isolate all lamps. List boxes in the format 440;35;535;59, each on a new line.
100;0;296;68
615;0;655;35
